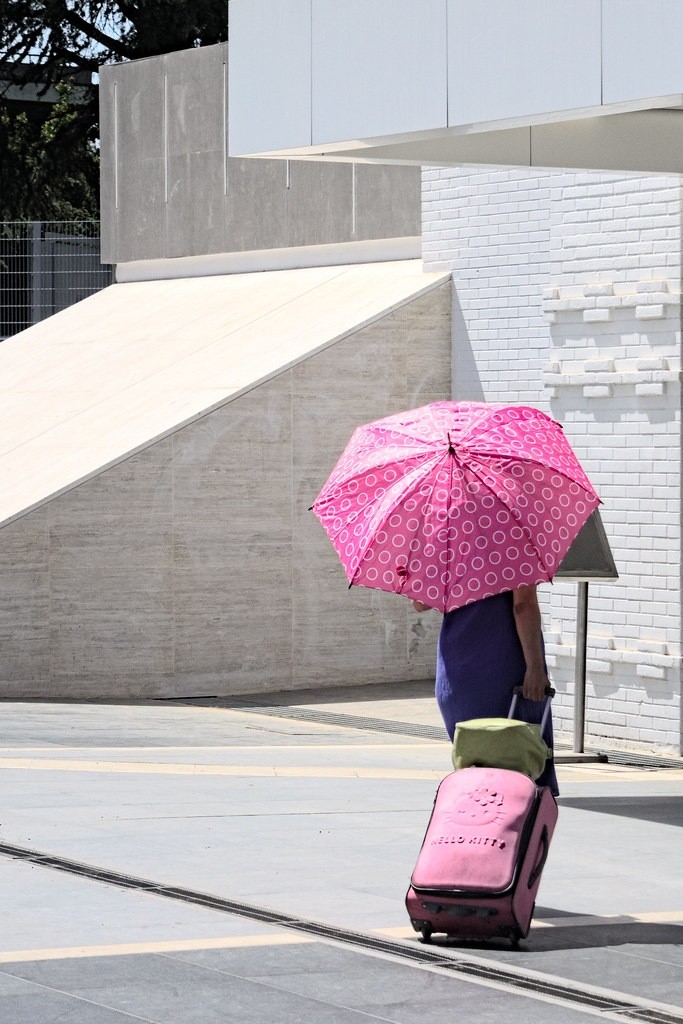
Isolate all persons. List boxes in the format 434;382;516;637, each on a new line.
413;585;559;798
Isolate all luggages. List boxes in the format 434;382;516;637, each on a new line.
406;685;560;943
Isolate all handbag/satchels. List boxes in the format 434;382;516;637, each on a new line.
451;718;555;781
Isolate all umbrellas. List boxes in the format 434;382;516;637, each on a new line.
308;401;604;612
